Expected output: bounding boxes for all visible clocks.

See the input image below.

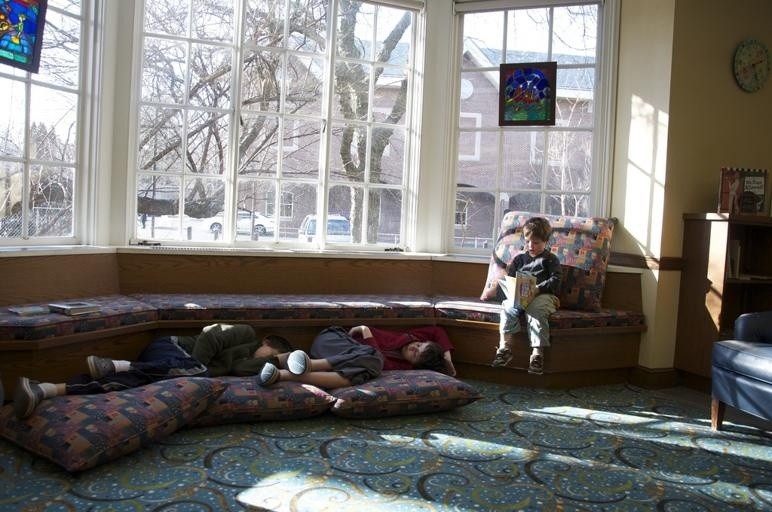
[731,40,771,94]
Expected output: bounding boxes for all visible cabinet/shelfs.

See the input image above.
[669,208,771,395]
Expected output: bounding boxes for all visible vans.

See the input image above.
[297,213,351,243]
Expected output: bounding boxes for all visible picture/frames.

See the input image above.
[1,0,51,75]
[496,60,558,127]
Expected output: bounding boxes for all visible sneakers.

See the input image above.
[256,361,280,387]
[14,376,45,418]
[85,354,116,380]
[527,353,545,375]
[490,347,514,368]
[286,349,310,376]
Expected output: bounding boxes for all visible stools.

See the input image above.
[703,307,771,437]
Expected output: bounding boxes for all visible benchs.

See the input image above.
[1,252,159,405]
[128,253,435,357]
[434,258,648,395]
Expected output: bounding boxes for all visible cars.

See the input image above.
[203,211,276,236]
[152,214,191,231]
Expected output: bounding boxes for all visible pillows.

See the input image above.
[0,365,229,478]
[321,360,486,422]
[480,208,617,315]
[185,369,339,425]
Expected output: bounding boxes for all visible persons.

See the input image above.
[492,217,562,376]
[12,324,293,419]
[256,325,456,386]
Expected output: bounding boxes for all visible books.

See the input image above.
[48,301,102,316]
[497,271,536,311]
